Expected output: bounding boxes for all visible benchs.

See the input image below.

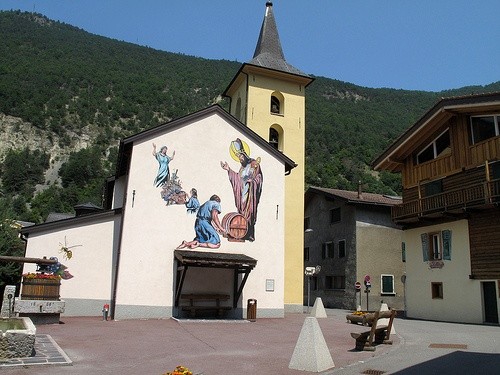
[351,308,397,351]
[181,294,232,318]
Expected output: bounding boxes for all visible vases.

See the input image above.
[21,278,62,300]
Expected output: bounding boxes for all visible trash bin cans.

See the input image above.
[247,299,257,322]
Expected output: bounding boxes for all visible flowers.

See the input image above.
[22,273,61,280]
[353,311,368,316]
[163,365,193,375]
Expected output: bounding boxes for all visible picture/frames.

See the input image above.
[266,279,274,291]
